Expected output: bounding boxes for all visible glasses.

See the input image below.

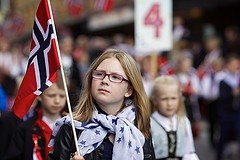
[92,69,129,83]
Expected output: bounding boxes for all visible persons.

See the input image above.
[149,74,200,160]
[223,25,240,58]
[0,5,225,111]
[5,70,70,160]
[163,51,202,139]
[50,49,156,160]
[199,54,225,149]
[213,54,240,160]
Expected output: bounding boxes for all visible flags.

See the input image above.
[62,0,85,15]
[3,11,26,36]
[11,0,62,120]
[93,0,114,12]
